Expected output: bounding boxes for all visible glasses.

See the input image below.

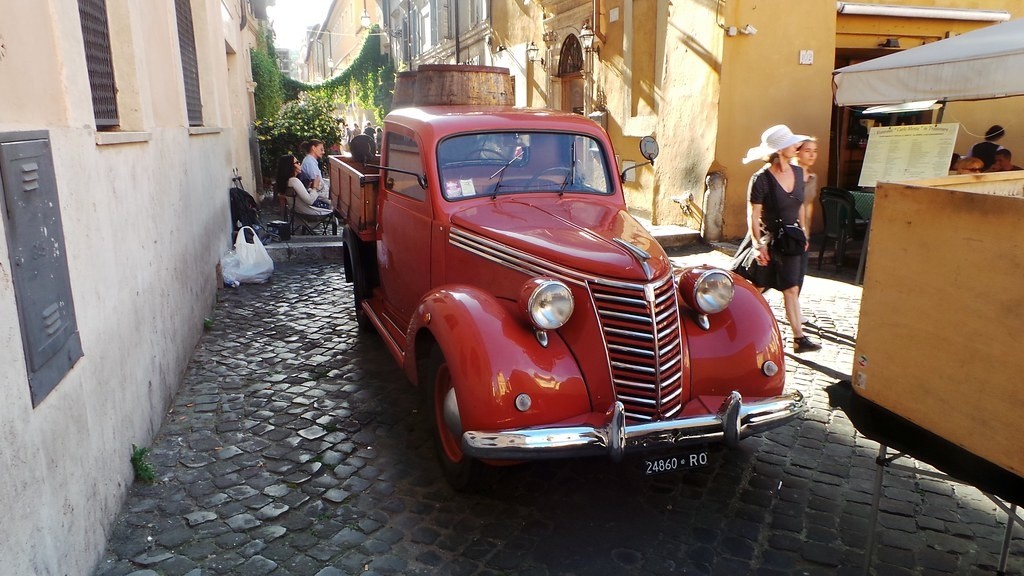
[294,160,299,164]
[963,168,984,172]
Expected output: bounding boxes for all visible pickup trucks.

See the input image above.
[326,105,809,498]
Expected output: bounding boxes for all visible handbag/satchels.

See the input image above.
[234,226,274,284]
[773,225,807,256]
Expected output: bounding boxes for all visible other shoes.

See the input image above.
[801,316,809,323]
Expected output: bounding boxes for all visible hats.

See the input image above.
[742,124,809,165]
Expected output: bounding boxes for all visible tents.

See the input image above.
[831,18,1024,107]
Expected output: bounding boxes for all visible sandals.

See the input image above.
[794,336,822,353]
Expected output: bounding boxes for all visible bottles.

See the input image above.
[222,251,240,287]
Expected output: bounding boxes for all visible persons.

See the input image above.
[274,139,350,228]
[341,122,383,155]
[949,125,1024,173]
[728,125,823,353]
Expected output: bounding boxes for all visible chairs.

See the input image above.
[272,184,337,236]
[818,186,872,286]
[849,189,875,285]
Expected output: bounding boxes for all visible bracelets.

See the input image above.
[312,187,318,190]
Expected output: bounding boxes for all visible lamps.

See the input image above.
[328,56,344,70]
[877,39,900,48]
[525,39,543,66]
[576,23,600,62]
[485,33,506,54]
[361,11,371,30]
[836,1,1011,22]
[317,73,325,82]
